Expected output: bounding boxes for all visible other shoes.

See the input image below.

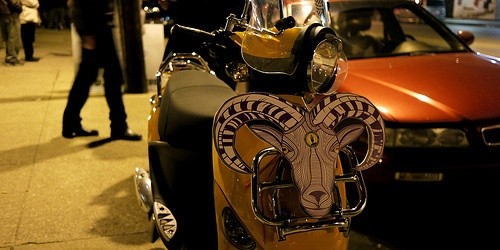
[25,57,39,61]
[5,58,23,65]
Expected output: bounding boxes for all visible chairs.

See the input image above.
[335,8,390,60]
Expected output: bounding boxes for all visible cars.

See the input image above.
[285,0,500,244]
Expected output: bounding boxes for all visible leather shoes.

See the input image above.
[62,126,98,137]
[111,129,141,140]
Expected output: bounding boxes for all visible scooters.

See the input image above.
[135,0,386,250]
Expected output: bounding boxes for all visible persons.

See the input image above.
[19,0,42,63]
[4,0,23,67]
[61,1,143,143]
